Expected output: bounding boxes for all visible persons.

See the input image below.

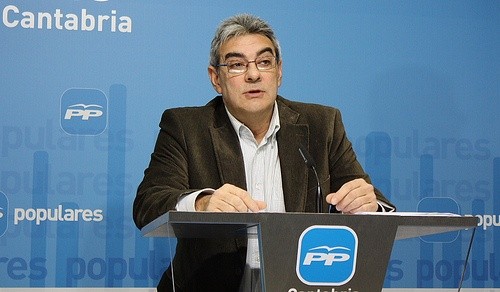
[132,12,398,292]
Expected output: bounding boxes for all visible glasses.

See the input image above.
[215,55,279,74]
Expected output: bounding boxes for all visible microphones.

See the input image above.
[298,145,314,167]
